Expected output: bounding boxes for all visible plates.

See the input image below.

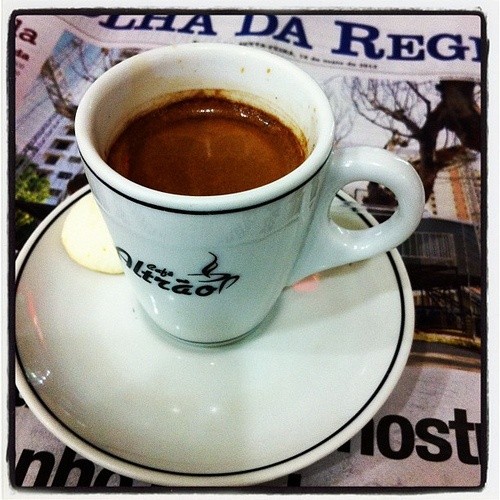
[14,183,416,487]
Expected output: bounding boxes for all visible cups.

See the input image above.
[73,43,425,349]
